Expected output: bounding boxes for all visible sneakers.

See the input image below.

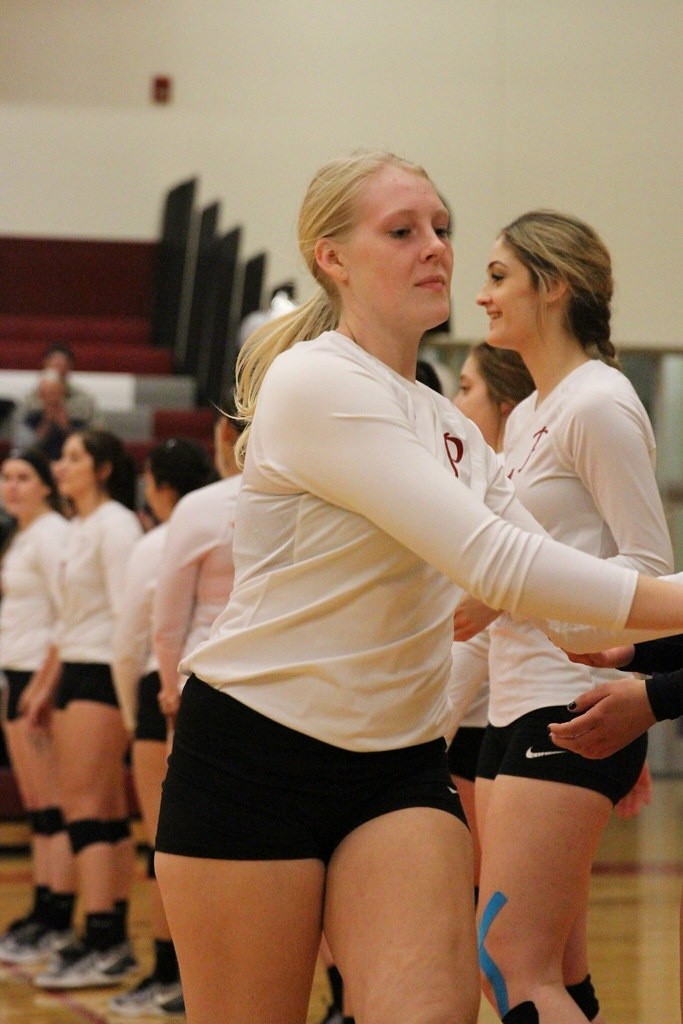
[0,919,81,967]
[112,979,185,1018]
[31,938,138,990]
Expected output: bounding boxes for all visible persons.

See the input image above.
[154,153,682,1024]
[546,633,683,759]
[11,344,103,459]
[415,213,672,1024]
[0,390,246,1014]
